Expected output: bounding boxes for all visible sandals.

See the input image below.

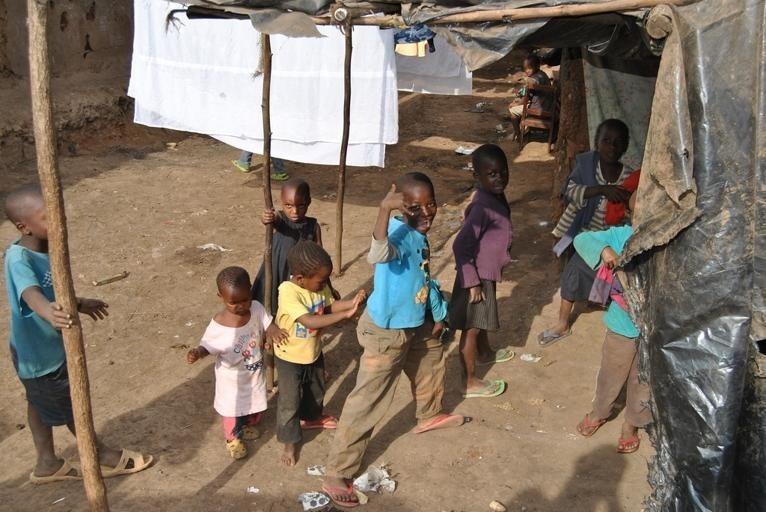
[231,160,250,172]
[270,173,289,181]
[226,437,248,459]
[239,425,260,440]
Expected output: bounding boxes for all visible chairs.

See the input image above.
[519,82,558,153]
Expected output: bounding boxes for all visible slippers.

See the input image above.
[100,447,153,478]
[29,458,84,485]
[576,414,606,436]
[321,483,359,507]
[539,330,572,348]
[618,433,639,452]
[475,349,514,367]
[300,414,338,429]
[414,413,465,433]
[462,378,505,398]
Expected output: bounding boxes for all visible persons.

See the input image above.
[230,150,292,181]
[537,118,638,349]
[321,170,465,508]
[447,145,515,398]
[572,188,656,453]
[507,55,554,142]
[186,265,292,461]
[2,184,154,485]
[249,179,342,385]
[272,239,368,467]
